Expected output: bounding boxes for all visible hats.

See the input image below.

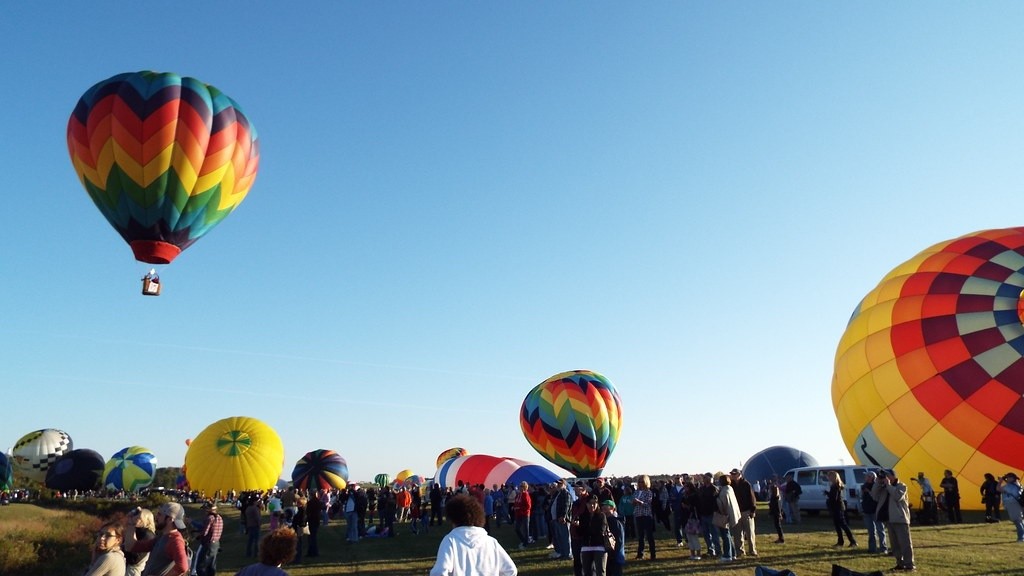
[702,473,712,478]
[161,502,186,529]
[863,470,877,479]
[556,479,566,485]
[597,478,605,483]
[730,469,739,474]
[571,481,586,487]
[201,501,214,509]
[879,469,896,477]
[601,500,616,508]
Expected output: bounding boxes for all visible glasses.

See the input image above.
[96,532,117,538]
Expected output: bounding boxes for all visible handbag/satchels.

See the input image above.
[600,513,616,552]
[1010,484,1024,507]
[712,503,728,528]
[685,507,703,535]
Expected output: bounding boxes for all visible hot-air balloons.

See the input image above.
[9,428,73,487]
[0,451,14,493]
[520,370,623,479]
[67,71,259,295]
[177,417,288,501]
[742,446,818,491]
[432,454,576,501]
[831,227,1024,511]
[436,448,467,470]
[375,474,390,488]
[390,470,425,488]
[45,449,104,493]
[102,446,156,496]
[347,481,360,490]
[292,449,348,490]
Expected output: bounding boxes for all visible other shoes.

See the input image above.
[889,565,916,572]
[835,542,843,547]
[516,536,573,560]
[674,542,757,564]
[775,538,784,543]
[848,542,858,548]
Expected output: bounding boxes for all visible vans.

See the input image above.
[782,465,881,516]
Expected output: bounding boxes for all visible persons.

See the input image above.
[940,470,962,523]
[997,474,1024,542]
[227,479,532,561]
[753,474,802,543]
[85,503,189,576]
[861,469,916,571]
[161,487,222,503]
[0,486,42,501]
[234,527,297,576]
[430,494,518,576]
[528,469,757,576]
[823,472,858,548]
[980,474,1001,519]
[51,488,150,501]
[917,474,938,520]
[198,502,223,576]
